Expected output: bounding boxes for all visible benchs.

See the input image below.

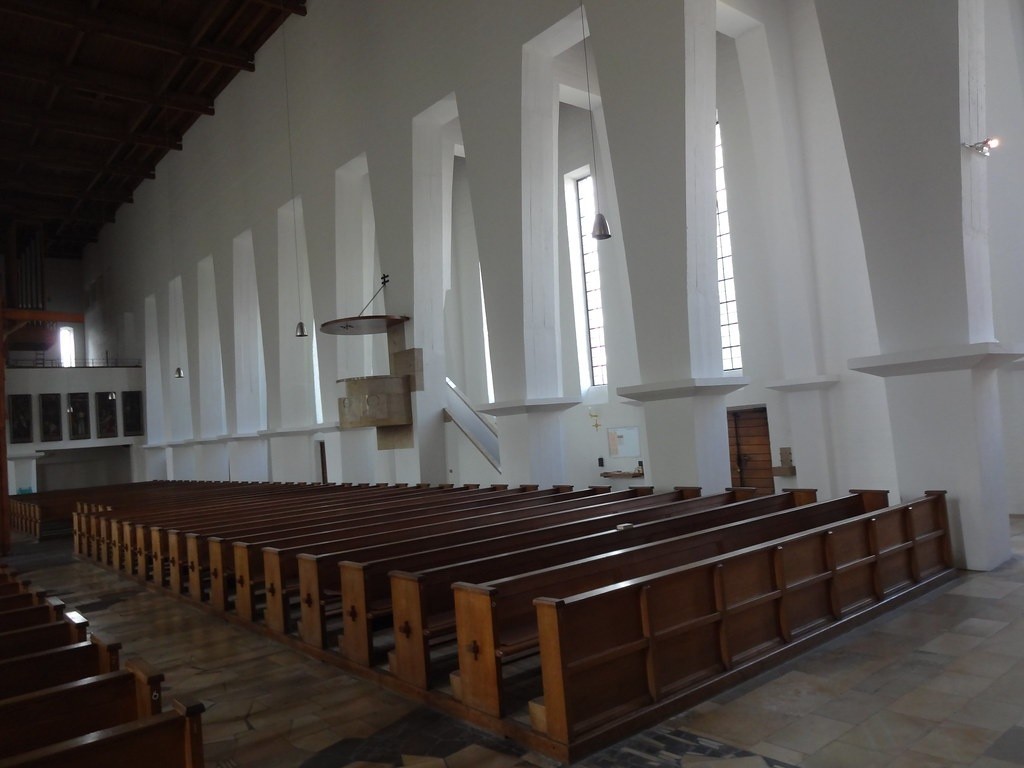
[0,479,960,768]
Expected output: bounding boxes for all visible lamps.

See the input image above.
[67,406,73,413]
[174,368,184,379]
[296,323,307,336]
[109,392,116,399]
[580,0,611,240]
